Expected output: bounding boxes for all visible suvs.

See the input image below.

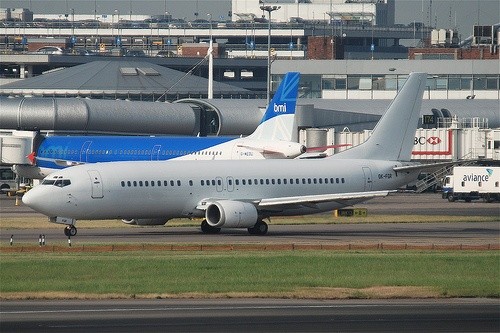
[122,49,146,57]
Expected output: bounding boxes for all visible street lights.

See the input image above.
[260,5,281,109]
[165,11,170,44]
[113,9,120,45]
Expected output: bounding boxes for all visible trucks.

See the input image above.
[440,166,500,203]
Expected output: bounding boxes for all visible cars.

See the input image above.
[188,18,209,28]
[154,50,175,57]
[31,45,95,56]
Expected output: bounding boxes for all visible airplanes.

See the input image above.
[8,71,308,181]
[21,71,494,237]
